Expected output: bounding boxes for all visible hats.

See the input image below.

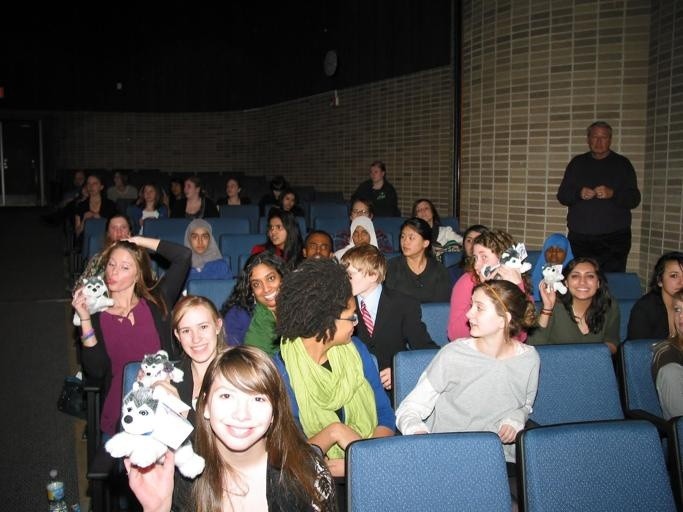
[270,175,289,191]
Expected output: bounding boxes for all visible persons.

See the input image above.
[270,257,395,511]
[71,236,191,445]
[652,289,683,421]
[124,344,338,512]
[136,294,231,421]
[392,279,540,511]
[447,224,682,350]
[52,162,244,279]
[221,163,464,396]
[556,121,642,274]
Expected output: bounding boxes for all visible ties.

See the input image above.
[360,300,373,338]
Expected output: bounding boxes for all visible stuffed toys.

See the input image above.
[104,379,204,479]
[140,350,183,383]
[73,276,115,326]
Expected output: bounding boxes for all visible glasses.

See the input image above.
[339,314,359,327]
[351,208,368,215]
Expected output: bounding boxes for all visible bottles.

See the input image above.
[45,468,69,511]
[66,489,80,512]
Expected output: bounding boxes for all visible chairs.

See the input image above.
[61,165,683,512]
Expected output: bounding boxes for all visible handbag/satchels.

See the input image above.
[58,375,86,419]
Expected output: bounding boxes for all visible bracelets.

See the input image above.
[79,317,90,322]
[78,328,95,344]
